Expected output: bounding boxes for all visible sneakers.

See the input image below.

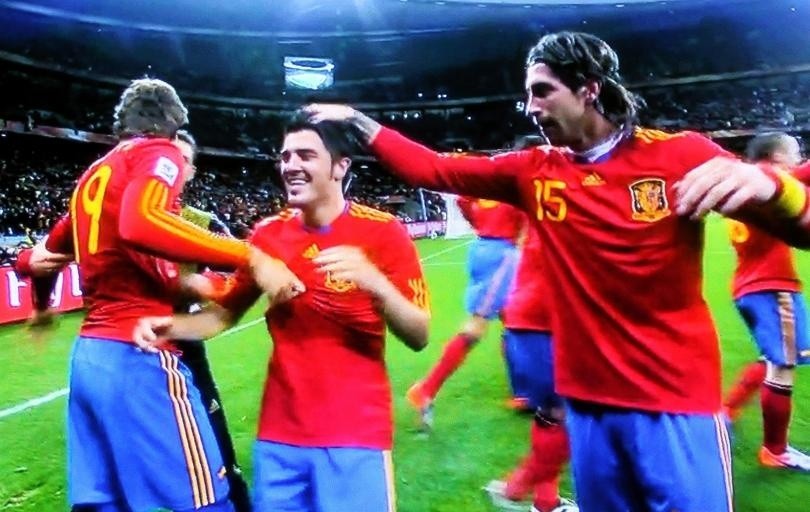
[758,441,809,471]
[529,494,579,512]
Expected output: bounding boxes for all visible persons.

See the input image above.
[0,72,115,235]
[31,78,305,511]
[309,32,810,512]
[644,87,808,163]
[407,137,577,511]
[184,137,286,238]
[343,162,447,223]
[17,131,253,512]
[722,132,809,470]
[133,109,431,512]
